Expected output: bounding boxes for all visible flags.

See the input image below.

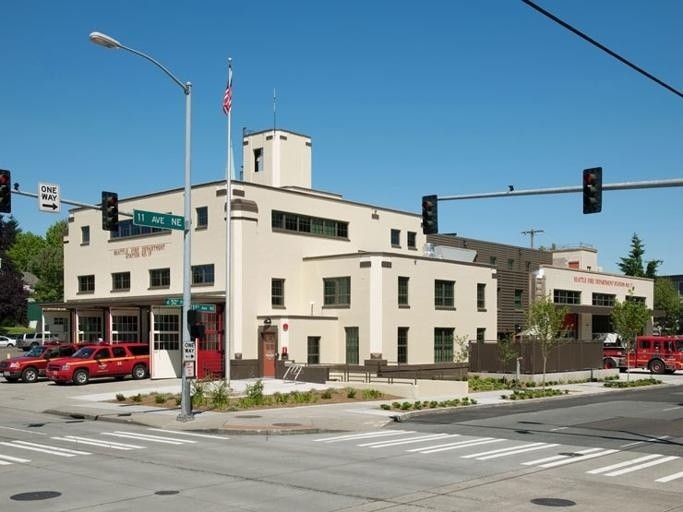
[223,64,232,116]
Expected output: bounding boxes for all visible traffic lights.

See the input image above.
[102,191,118,231]
[583,167,602,214]
[0,169,10,213]
[422,195,438,234]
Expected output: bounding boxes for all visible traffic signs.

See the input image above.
[38,182,60,213]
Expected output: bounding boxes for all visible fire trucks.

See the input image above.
[603,335,683,374]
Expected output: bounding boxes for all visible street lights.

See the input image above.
[89,32,192,416]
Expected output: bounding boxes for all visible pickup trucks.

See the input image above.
[0,331,149,385]
